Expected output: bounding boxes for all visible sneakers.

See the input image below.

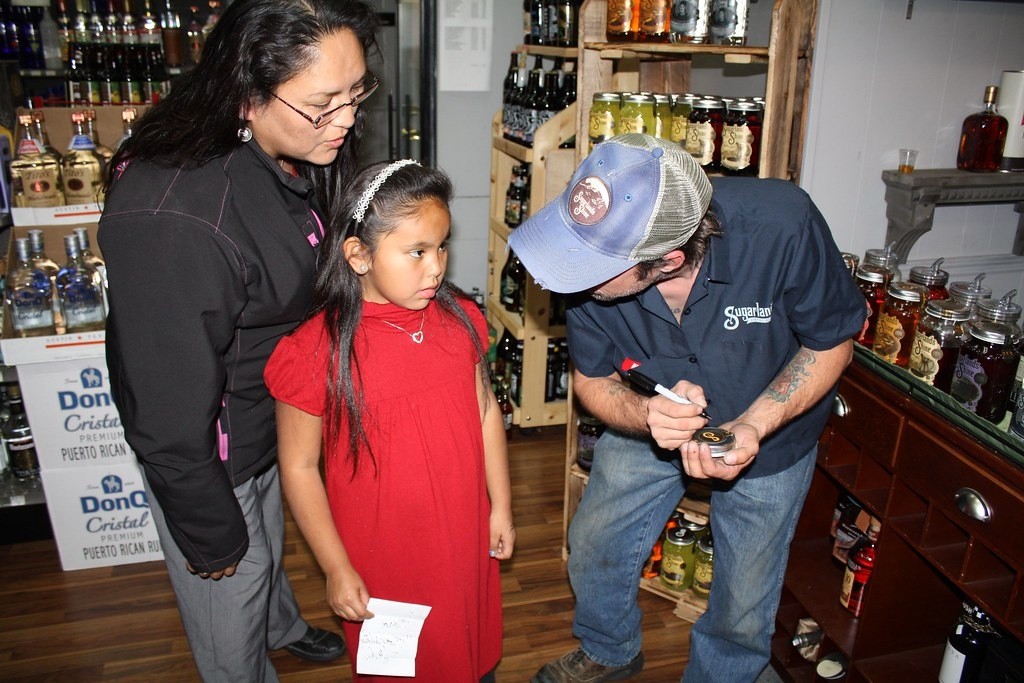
[530,646,645,683]
[286,625,346,662]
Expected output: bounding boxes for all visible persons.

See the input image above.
[96,0,386,682]
[263,159,516,683]
[508,132,867,683]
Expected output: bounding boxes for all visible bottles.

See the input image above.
[829,493,883,622]
[0,0,219,340]
[576,414,714,599]
[484,0,767,440]
[0,381,40,477]
[956,86,1008,174]
[938,605,1002,683]
[837,248,1024,441]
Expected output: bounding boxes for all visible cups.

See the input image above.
[899,148,918,174]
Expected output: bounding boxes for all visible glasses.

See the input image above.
[261,71,381,130]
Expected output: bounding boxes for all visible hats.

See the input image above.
[506,132,715,295]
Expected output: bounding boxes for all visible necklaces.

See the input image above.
[382,311,425,344]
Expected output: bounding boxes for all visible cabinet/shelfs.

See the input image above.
[0,0,1024,683]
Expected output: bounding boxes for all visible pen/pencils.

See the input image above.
[626,369,711,419]
